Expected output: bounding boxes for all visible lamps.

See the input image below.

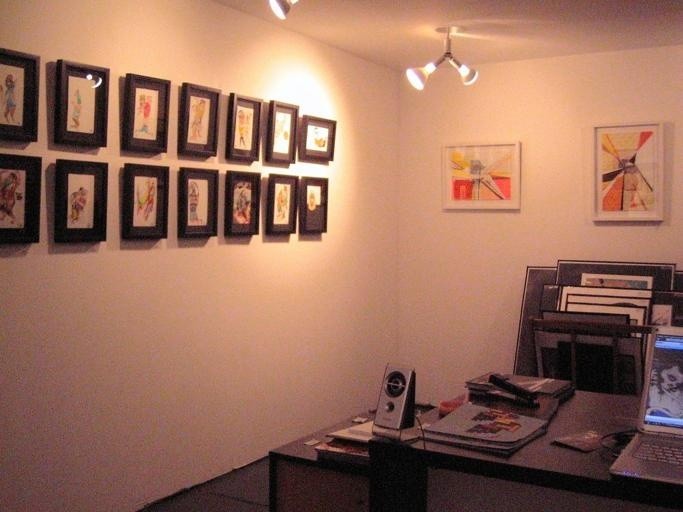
[403,24,478,90]
[267,0,302,19]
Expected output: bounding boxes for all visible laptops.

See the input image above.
[609,325,683,486]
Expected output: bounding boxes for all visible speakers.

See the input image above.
[372,363,418,441]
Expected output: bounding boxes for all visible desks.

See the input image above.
[266,406,435,512]
[366,375,682,511]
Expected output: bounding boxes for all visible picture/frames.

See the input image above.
[56,59,109,146]
[266,101,298,162]
[180,82,220,155]
[300,115,332,160]
[224,170,262,235]
[0,46,40,142]
[2,154,41,243]
[515,258,683,395]
[123,74,170,152]
[227,93,262,161]
[123,163,168,238]
[55,159,108,243]
[440,141,521,210]
[300,175,329,233]
[267,173,298,233]
[591,120,664,222]
[178,167,219,236]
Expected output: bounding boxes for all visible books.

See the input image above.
[465,372,577,408]
[314,420,375,465]
